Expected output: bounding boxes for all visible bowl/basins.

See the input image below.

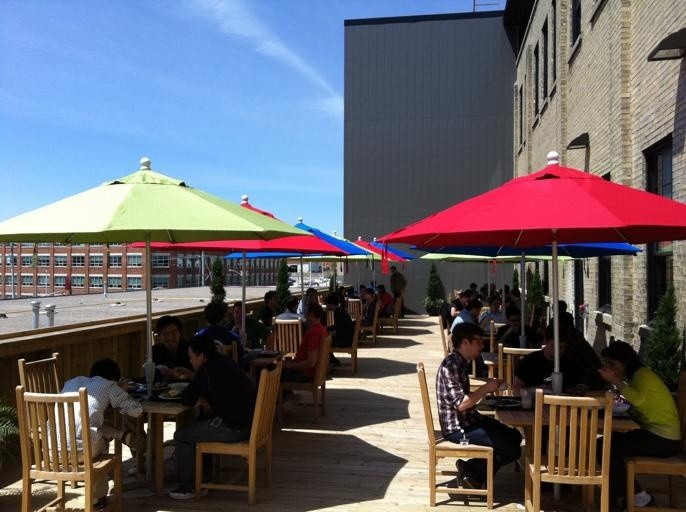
[167,382,188,391]
[612,403,630,413]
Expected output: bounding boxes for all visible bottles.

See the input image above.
[520,389,535,408]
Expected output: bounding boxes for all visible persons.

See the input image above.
[180,336,253,500]
[277,304,329,420]
[360,280,395,336]
[513,327,575,393]
[152,315,196,371]
[195,299,266,351]
[436,323,522,491]
[256,291,282,326]
[324,295,354,368]
[450,285,573,347]
[390,268,407,318]
[592,341,681,509]
[44,358,144,465]
[276,297,304,319]
[303,288,318,307]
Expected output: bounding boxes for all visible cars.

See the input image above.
[286,276,342,287]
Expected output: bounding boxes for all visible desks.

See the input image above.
[116,379,191,499]
[492,390,640,504]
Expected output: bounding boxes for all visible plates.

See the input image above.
[157,391,180,400]
[260,350,276,354]
[128,394,146,401]
[486,395,522,408]
[140,384,166,392]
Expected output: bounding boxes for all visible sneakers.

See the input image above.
[169,486,207,500]
[126,434,146,451]
[456,459,484,490]
[633,490,652,507]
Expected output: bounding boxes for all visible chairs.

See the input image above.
[524,387,615,512]
[264,286,402,369]
[418,360,495,511]
[15,384,123,512]
[627,325,685,512]
[15,352,64,440]
[418,288,686,402]
[195,361,284,504]
[278,335,332,425]
